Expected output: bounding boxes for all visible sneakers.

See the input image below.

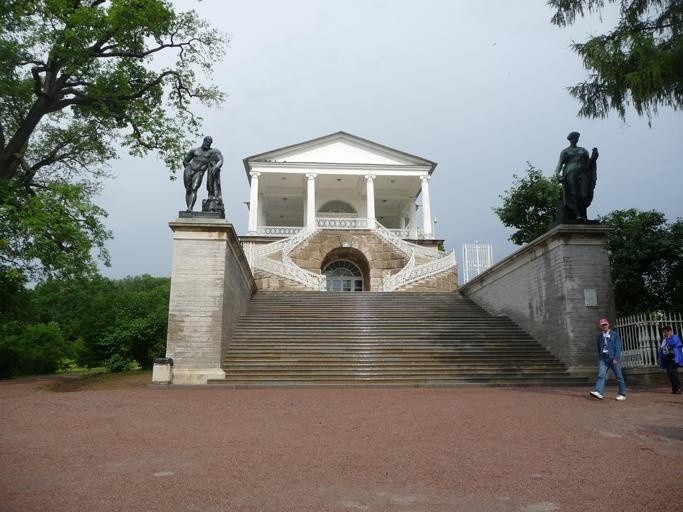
[616,394,625,400]
[590,392,603,399]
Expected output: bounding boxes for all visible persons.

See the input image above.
[556,131,599,221]
[182,136,223,212]
[657,325,683,394]
[590,317,628,400]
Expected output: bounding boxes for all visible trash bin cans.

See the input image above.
[152,358,173,384]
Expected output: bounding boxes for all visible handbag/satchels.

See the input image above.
[661,345,675,361]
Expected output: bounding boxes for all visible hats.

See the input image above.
[599,319,609,325]
